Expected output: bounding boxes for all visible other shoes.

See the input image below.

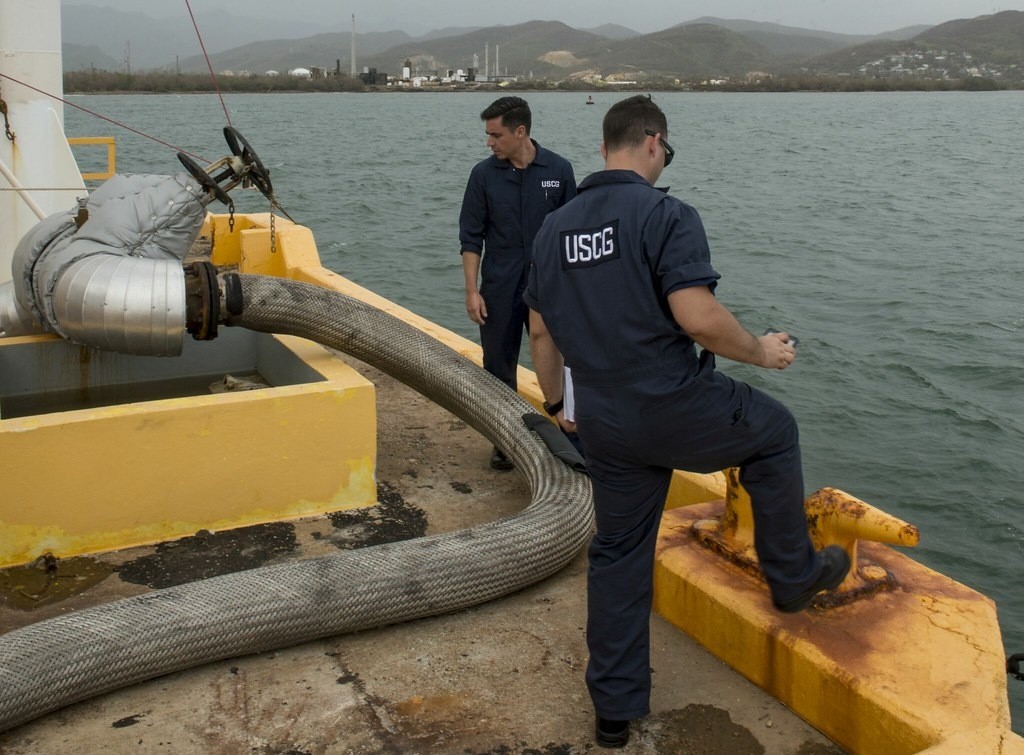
[596,712,631,748]
[774,545,851,615]
[491,444,515,469]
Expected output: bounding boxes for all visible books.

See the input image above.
[561,362,574,426]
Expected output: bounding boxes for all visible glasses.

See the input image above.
[646,128,675,167]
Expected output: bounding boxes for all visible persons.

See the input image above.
[458,96,578,471]
[521,94,853,749]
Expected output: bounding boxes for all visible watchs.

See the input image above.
[540,398,564,418]
